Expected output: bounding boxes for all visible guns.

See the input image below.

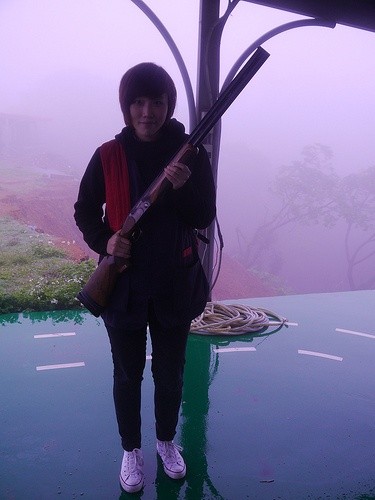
[75,45,271,318]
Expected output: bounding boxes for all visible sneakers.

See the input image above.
[118,447,145,494]
[155,436,187,479]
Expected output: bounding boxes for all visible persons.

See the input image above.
[70,60,216,495]
